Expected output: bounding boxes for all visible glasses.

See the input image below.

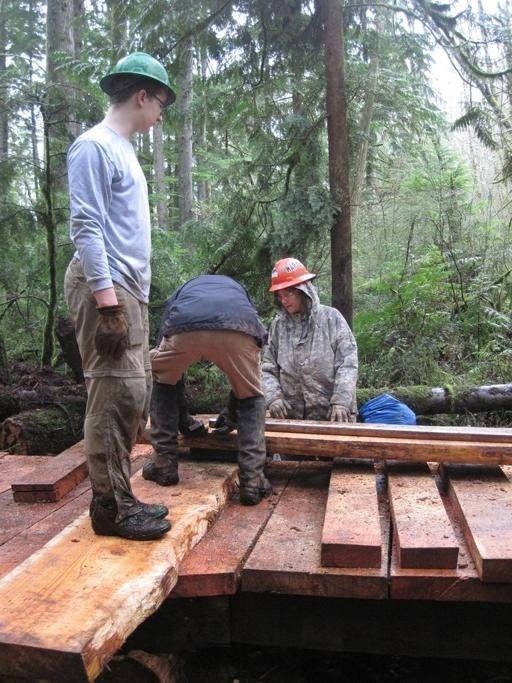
[272,289,297,298]
[154,95,169,111]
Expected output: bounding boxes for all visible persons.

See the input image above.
[262,258,358,422]
[63,51,172,539]
[143,276,272,505]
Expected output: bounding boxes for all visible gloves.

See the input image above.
[181,410,207,437]
[325,405,353,423]
[268,398,293,419]
[94,305,131,361]
[212,405,235,435]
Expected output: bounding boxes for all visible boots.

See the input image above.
[230,389,272,506]
[142,377,185,486]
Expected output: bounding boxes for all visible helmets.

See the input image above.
[99,52,176,107]
[268,258,317,292]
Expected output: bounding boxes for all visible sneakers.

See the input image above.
[89,502,171,540]
